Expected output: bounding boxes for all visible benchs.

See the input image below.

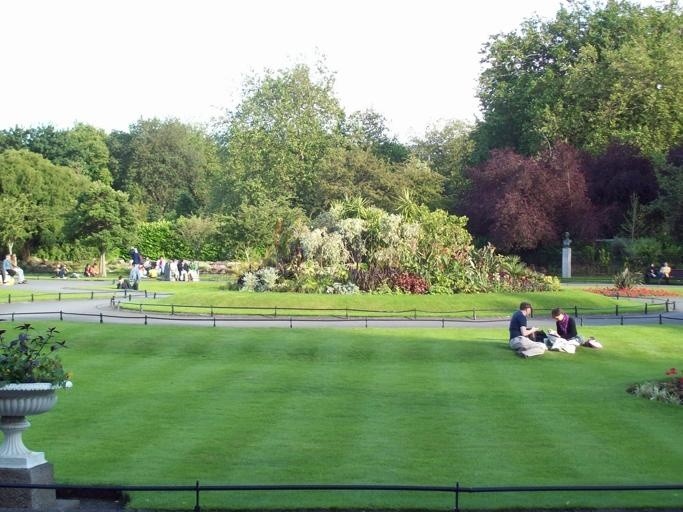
[643,268,683,286]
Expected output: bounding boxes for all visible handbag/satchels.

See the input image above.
[535,331,548,342]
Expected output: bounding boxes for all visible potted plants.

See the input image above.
[0,318,73,468]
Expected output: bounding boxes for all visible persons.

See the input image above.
[659,261,671,286]
[548,308,583,346]
[646,263,657,283]
[2,254,15,277]
[510,302,548,359]
[11,254,28,284]
[55,249,199,290]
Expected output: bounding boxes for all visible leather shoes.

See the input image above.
[515,352,527,358]
